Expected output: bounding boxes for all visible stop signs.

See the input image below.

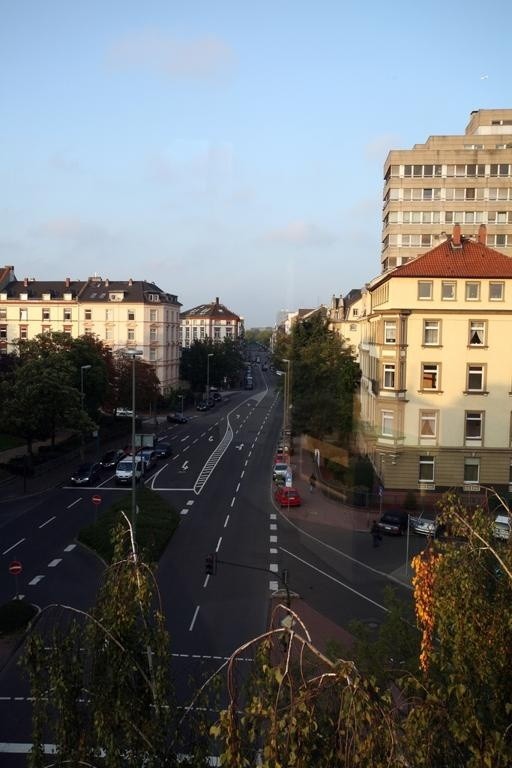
[92,495,103,504]
[9,561,22,575]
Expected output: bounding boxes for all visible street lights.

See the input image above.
[81,364,92,459]
[277,358,290,464]
[122,349,143,558]
[207,354,215,399]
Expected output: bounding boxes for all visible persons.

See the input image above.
[437,523,447,542]
[370,520,381,547]
[309,473,316,492]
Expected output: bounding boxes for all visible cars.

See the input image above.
[68,463,103,487]
[100,448,126,466]
[378,509,407,536]
[197,393,221,411]
[137,442,170,469]
[256,357,268,371]
[273,425,301,507]
[243,364,253,390]
[167,415,187,423]
[409,514,435,537]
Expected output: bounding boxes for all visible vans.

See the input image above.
[115,456,145,484]
[493,515,512,540]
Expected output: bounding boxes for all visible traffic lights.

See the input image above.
[206,553,217,576]
